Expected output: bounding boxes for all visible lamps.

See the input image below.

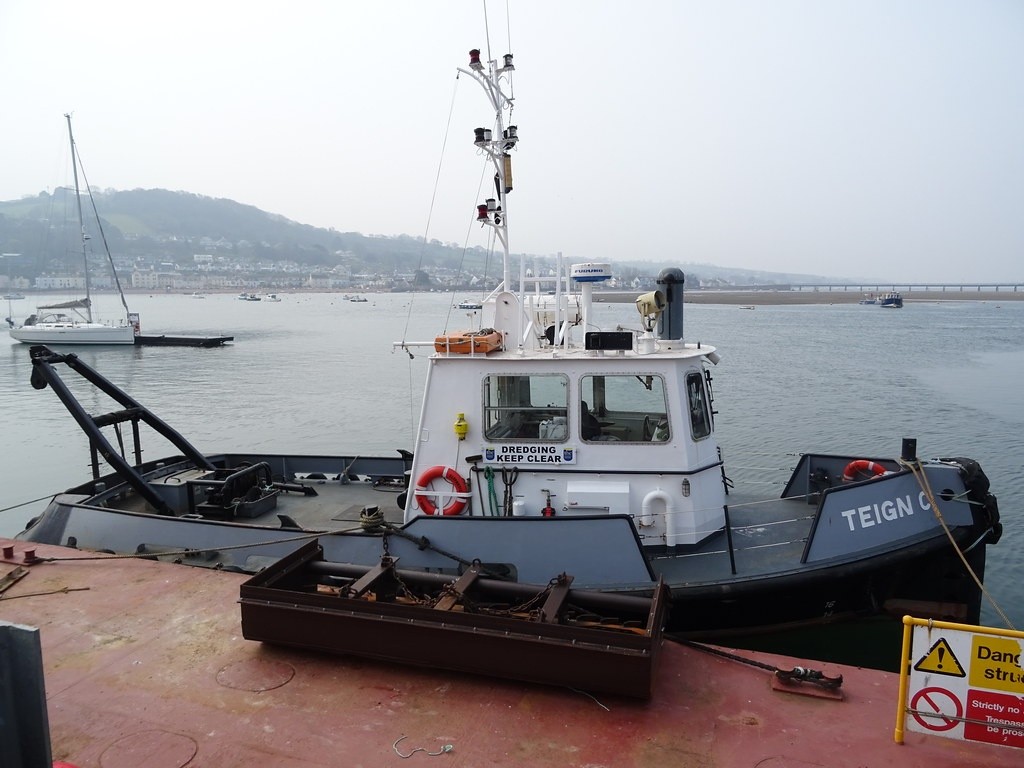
[636,290,667,332]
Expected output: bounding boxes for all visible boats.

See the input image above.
[458,298,481,310]
[238,288,282,304]
[349,297,368,303]
[858,294,878,305]
[7,2,1004,675]
[879,287,904,309]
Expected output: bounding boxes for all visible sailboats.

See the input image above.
[3,111,237,348]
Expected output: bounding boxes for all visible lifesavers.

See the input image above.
[415,466,468,516]
[842,460,887,482]
